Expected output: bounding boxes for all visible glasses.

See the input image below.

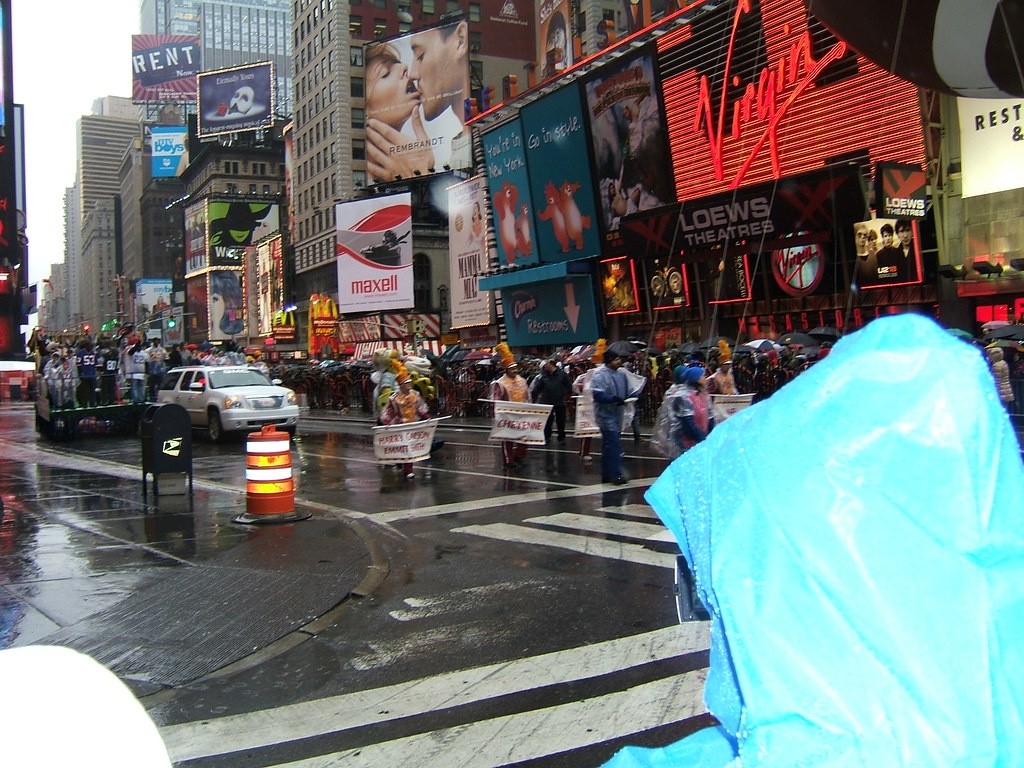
[856,232,868,239]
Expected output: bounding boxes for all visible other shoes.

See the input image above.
[406,473,415,478]
[601,475,626,485]
[579,456,593,461]
[393,466,397,468]
[50,398,158,411]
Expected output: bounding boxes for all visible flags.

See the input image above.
[20,283,39,314]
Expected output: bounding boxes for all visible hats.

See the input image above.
[820,341,832,347]
[396,373,412,384]
[603,349,619,366]
[717,355,733,364]
[102,339,113,347]
[128,337,140,345]
[187,344,200,351]
[46,342,59,351]
[495,343,518,368]
[209,348,224,354]
[592,353,603,364]
[66,350,73,353]
[200,339,213,351]
[674,365,704,384]
[152,338,160,343]
[253,350,263,357]
[246,356,255,361]
[779,346,789,353]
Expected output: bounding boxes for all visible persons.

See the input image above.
[854,219,917,284]
[599,313,1023,768]
[365,21,469,180]
[608,92,658,214]
[471,202,481,235]
[153,296,169,313]
[38,333,1024,492]
[210,270,243,338]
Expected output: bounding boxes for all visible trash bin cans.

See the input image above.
[140,403,194,497]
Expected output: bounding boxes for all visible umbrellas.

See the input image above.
[428,321,1024,367]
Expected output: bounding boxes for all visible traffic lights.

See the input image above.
[167,313,178,330]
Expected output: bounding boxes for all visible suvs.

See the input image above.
[155,364,301,445]
